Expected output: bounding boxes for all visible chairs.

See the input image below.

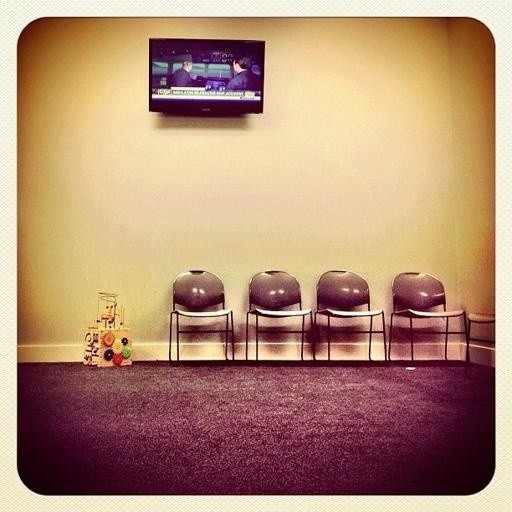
[245,271,316,368]
[169,271,235,369]
[313,272,388,370]
[466,312,495,372]
[388,272,471,368]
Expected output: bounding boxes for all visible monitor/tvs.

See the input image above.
[149,38,265,115]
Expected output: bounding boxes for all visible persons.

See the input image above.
[171,60,193,87]
[227,56,261,91]
